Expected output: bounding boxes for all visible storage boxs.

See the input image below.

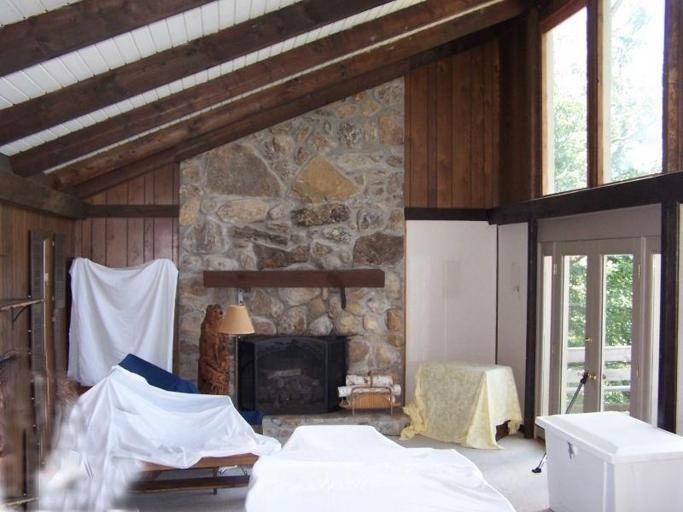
[535,411,683,512]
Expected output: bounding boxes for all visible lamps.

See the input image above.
[215,304,255,412]
